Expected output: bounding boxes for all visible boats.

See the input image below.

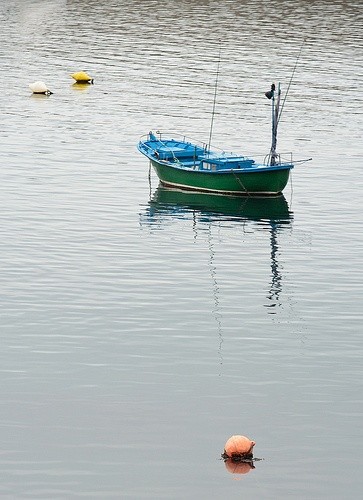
[142,187,293,313]
[139,39,313,198]
